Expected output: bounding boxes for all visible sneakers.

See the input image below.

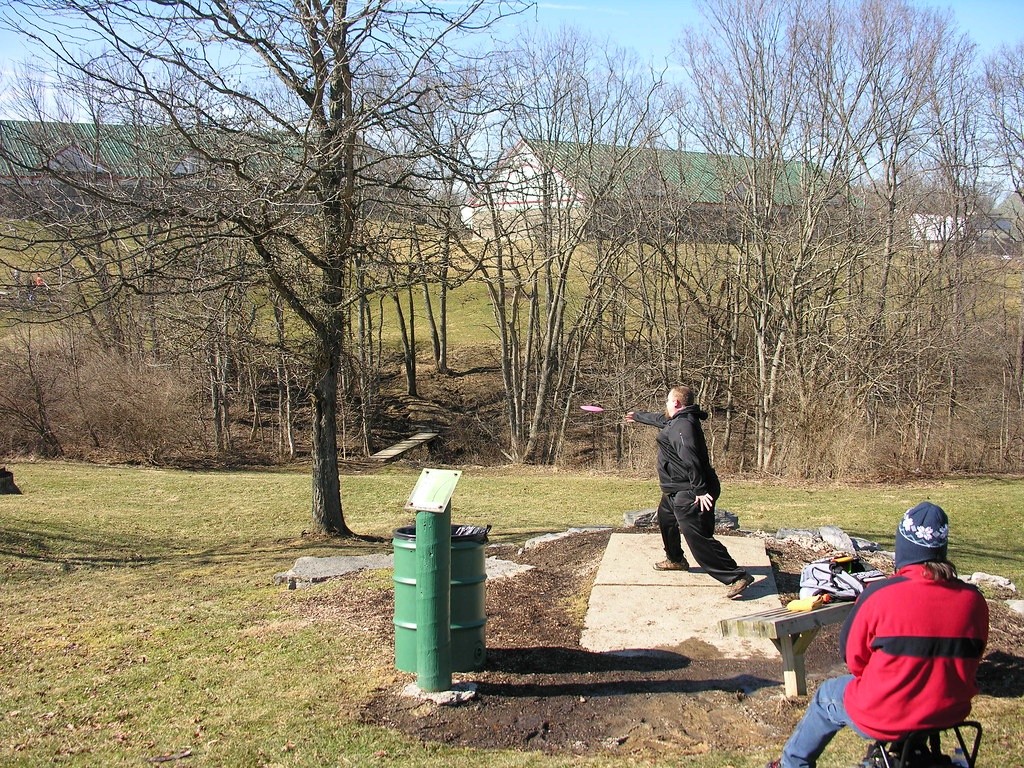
[653,557,689,571]
[727,573,755,598]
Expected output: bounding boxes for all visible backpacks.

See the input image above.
[799,555,887,602]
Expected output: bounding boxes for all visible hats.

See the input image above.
[895,501,948,569]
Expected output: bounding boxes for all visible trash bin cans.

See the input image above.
[392,527,490,670]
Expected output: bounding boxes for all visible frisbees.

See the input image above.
[580,405,604,412]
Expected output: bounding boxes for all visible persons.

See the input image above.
[765,501,989,768]
[625,386,755,598]
